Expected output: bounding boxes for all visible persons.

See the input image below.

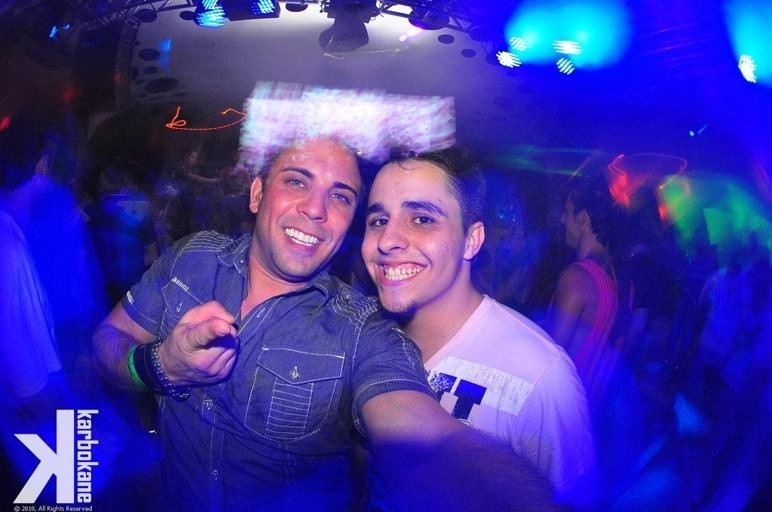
[0,118,770,510]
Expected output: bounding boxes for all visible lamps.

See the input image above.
[318,0,384,58]
